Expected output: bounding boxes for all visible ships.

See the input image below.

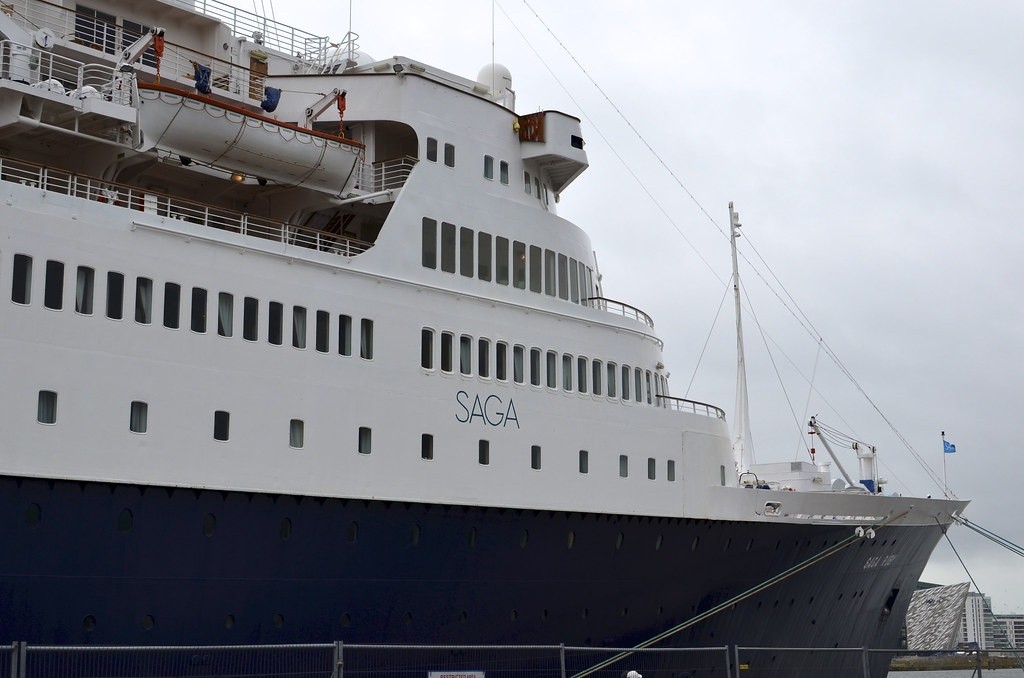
[1,3,975,678]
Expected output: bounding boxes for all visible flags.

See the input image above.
[944,441,956,453]
